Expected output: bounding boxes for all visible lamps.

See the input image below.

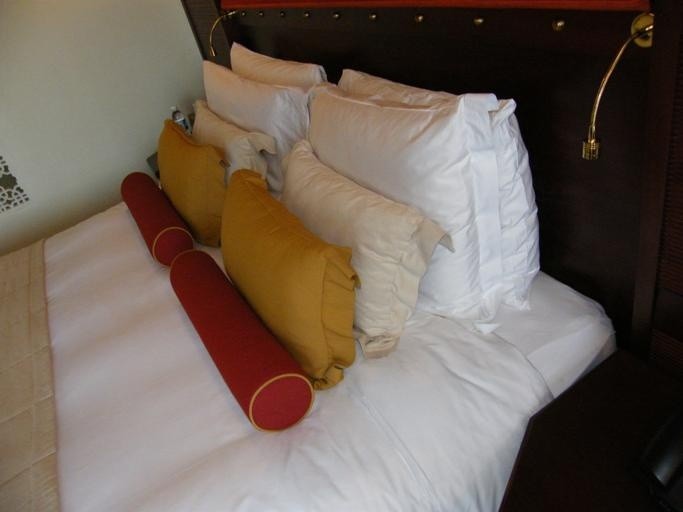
[202,8,237,58]
[575,11,655,163]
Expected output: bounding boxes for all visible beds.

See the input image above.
[0,3,676,512]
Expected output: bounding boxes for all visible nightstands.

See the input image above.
[501,342,679,512]
[144,149,161,182]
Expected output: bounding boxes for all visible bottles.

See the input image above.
[169,105,191,136]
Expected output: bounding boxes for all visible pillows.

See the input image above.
[219,168,362,395]
[200,56,308,191]
[305,80,482,322]
[165,247,315,433]
[152,118,234,247]
[332,65,546,323]
[188,95,278,181]
[118,168,195,268]
[228,39,327,96]
[275,136,458,354]
